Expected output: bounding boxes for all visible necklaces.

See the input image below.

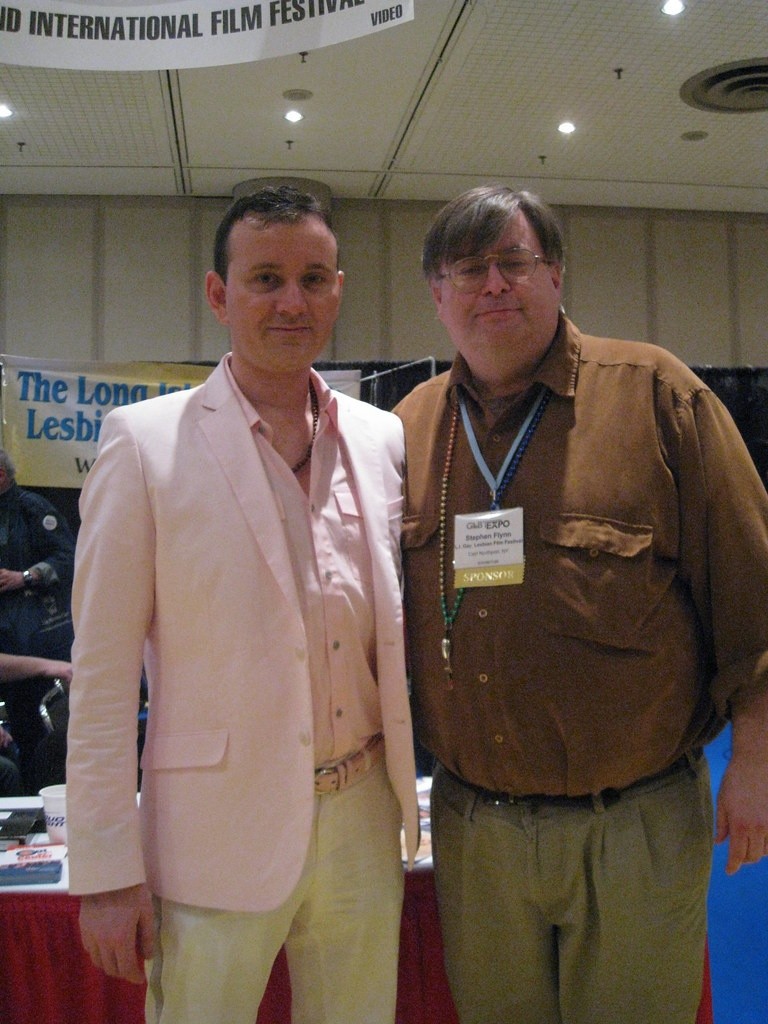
[439,389,552,690]
[290,378,317,473]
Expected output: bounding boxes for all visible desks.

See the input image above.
[0,781,712,1024]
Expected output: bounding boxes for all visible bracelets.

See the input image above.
[23,570,32,585]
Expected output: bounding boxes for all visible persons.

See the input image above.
[0,450,76,795]
[395,185,768,1024]
[0,653,72,797]
[69,186,422,1023]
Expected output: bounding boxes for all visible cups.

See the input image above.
[39,783,67,845]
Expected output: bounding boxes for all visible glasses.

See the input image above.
[438,249,552,295]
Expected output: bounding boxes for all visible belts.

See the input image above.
[437,742,704,811]
[315,733,386,798]
